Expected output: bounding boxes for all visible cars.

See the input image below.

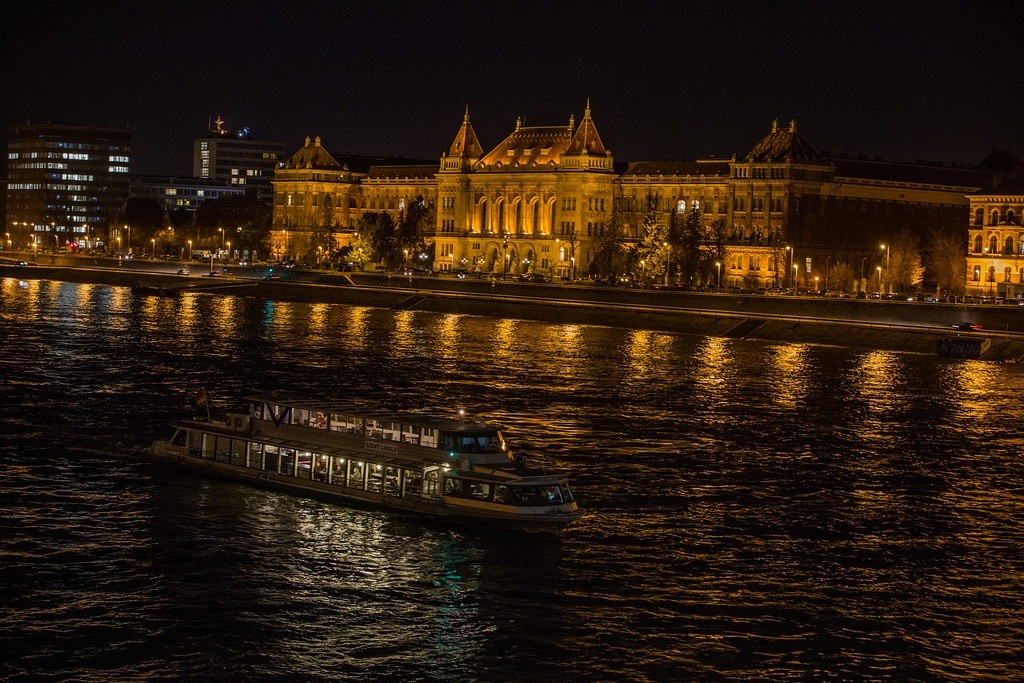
[270,258,1023,310]
[954,323,980,332]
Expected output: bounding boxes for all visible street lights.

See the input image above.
[571,257,574,281]
[188,240,192,258]
[794,264,799,293]
[218,227,224,249]
[404,249,408,274]
[880,244,890,293]
[355,231,361,253]
[282,229,289,260]
[419,253,428,273]
[4,224,91,253]
[664,242,671,276]
[462,258,468,275]
[715,263,720,291]
[877,266,882,300]
[117,237,121,250]
[168,227,174,248]
[786,245,793,287]
[226,242,231,256]
[151,239,155,257]
[984,239,995,304]
[640,260,645,273]
[522,258,531,277]
[124,224,131,250]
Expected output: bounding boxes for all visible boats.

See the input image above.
[143,388,588,537]
